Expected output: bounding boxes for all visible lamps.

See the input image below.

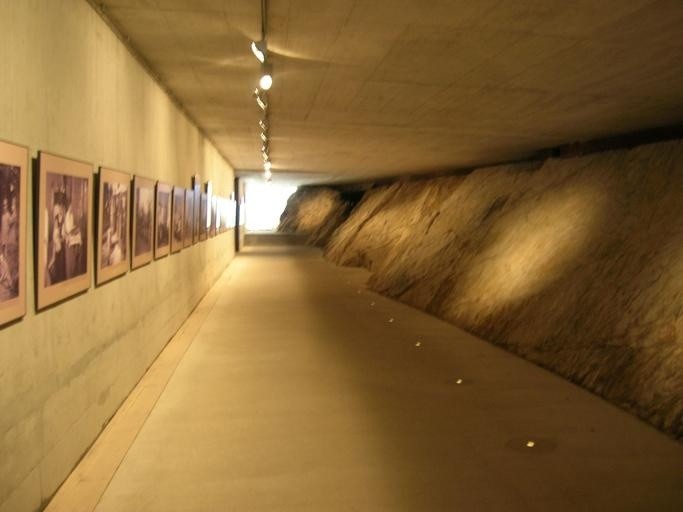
[250,40,273,181]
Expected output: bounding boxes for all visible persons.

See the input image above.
[1,195,11,252]
[47,191,81,286]
[6,196,19,284]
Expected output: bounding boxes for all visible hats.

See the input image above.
[54,191,66,207]
